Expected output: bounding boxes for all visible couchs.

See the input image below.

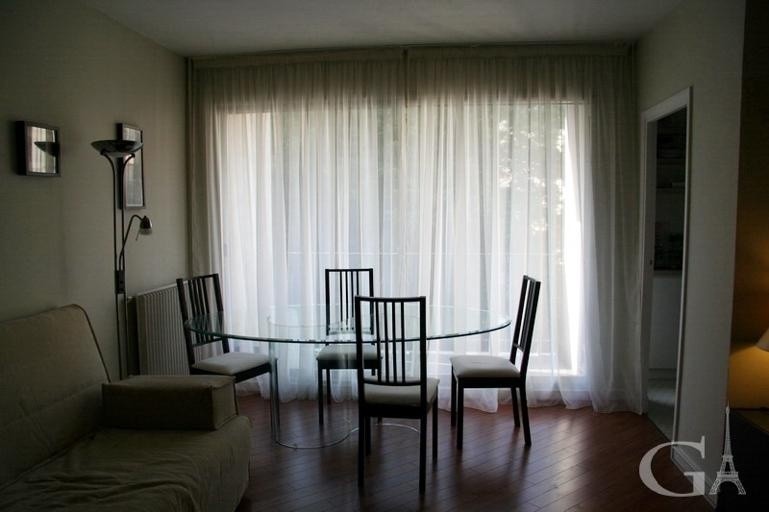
[1,303,249,512]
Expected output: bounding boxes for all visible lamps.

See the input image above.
[90,140,153,379]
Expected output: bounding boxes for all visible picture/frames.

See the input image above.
[21,120,62,177]
[117,123,147,210]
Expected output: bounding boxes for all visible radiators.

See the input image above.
[127,279,205,374]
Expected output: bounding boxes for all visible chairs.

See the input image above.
[176,269,543,499]
